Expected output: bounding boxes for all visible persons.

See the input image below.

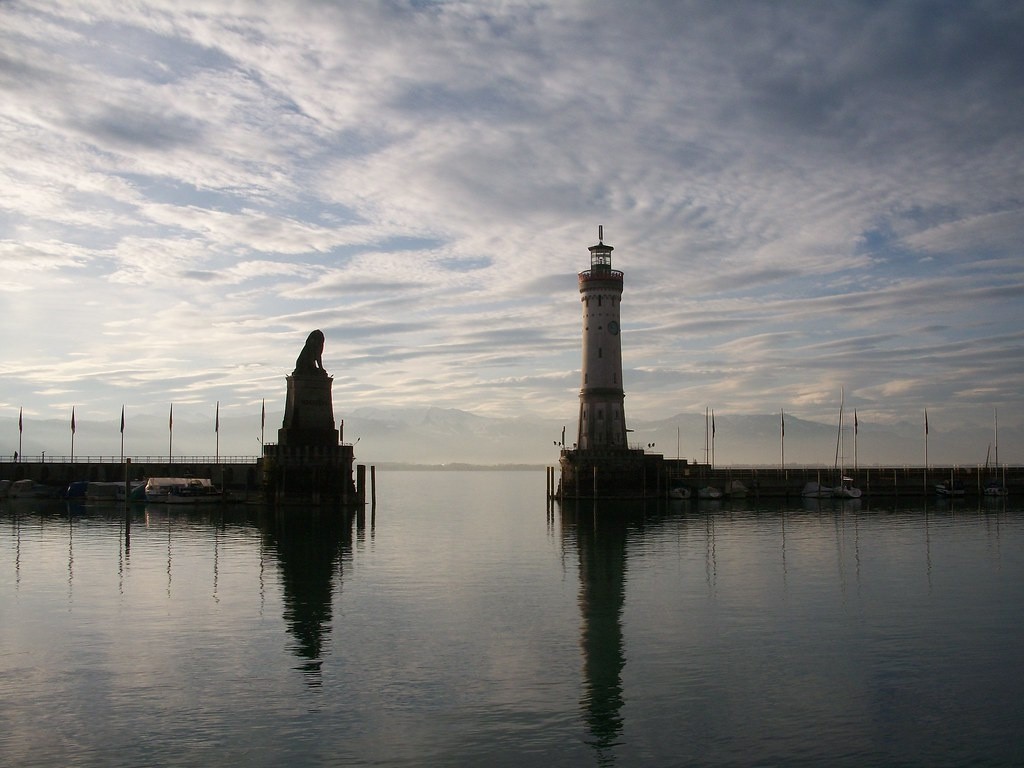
[14,451,18,461]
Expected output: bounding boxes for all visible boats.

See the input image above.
[1,479,12,499]
[670,478,692,500]
[9,478,49,498]
[801,481,834,498]
[698,484,723,501]
[984,484,1009,497]
[722,480,750,499]
[66,476,236,503]
[934,484,967,496]
[835,485,862,498]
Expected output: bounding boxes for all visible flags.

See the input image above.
[120,405,124,434]
[71,406,75,435]
[19,408,22,433]
